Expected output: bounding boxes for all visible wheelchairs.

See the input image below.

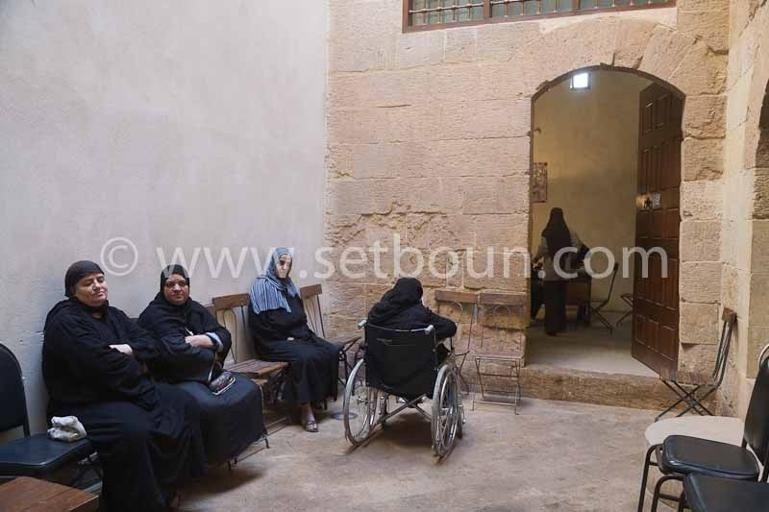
[339,312,466,456]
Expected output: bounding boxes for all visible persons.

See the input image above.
[532,207,579,336]
[248,247,338,432]
[367,278,457,409]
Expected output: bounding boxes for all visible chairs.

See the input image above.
[582,262,620,334]
[637,306,768,512]
[0,282,364,512]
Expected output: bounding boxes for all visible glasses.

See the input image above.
[278,259,293,266]
[165,279,188,287]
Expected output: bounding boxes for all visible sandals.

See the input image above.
[300,409,320,433]
